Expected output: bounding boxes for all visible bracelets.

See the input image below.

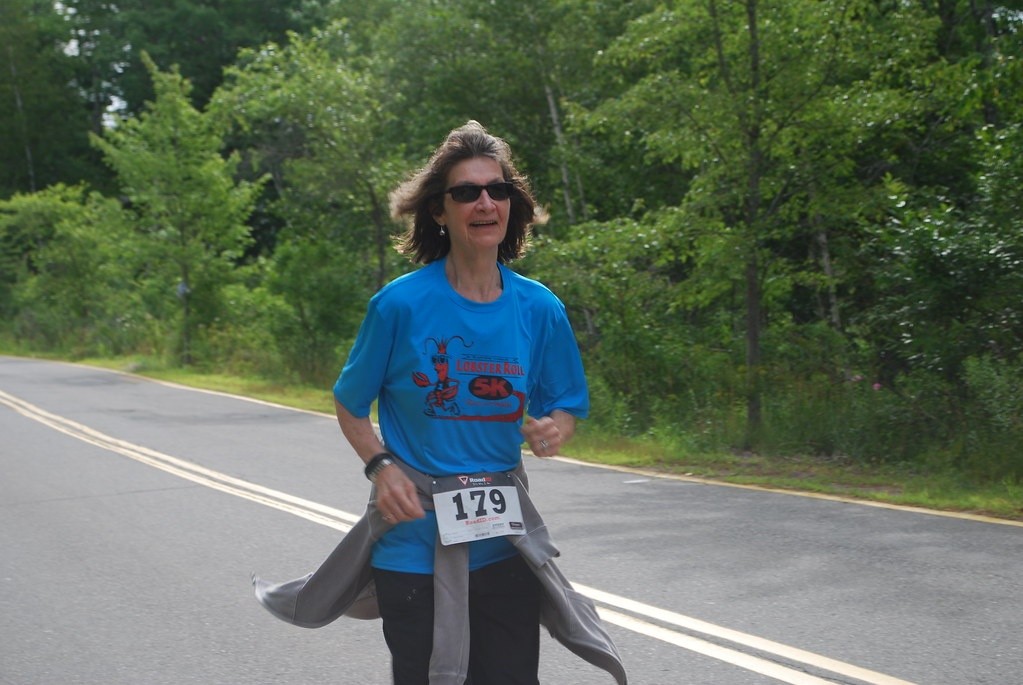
[365,453,394,483]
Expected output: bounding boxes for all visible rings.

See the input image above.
[540,440,549,449]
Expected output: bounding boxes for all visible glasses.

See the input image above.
[443,182,514,203]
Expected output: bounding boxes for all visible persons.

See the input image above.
[332,119,591,685]
